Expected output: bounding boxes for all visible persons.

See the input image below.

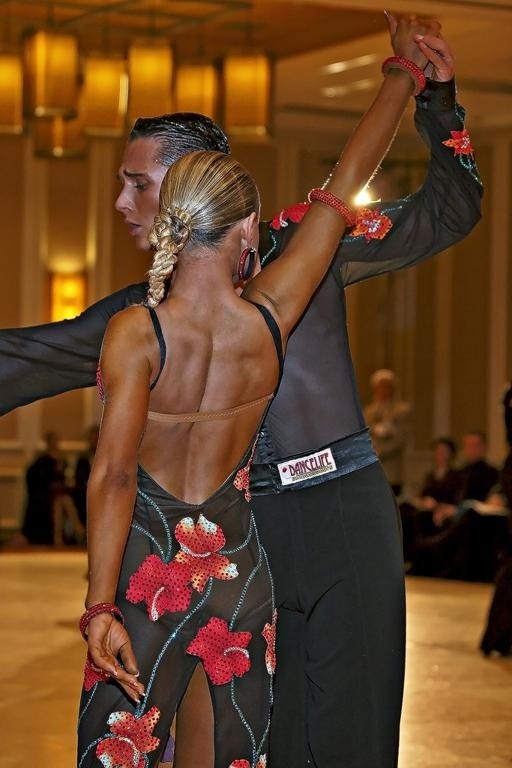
[23,426,85,547]
[480,390,511,660]
[76,8,443,768]
[395,438,465,573]
[73,426,101,528]
[362,366,414,503]
[0,29,488,766]
[414,478,512,584]
[405,431,500,578]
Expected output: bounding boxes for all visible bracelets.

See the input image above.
[382,55,424,98]
[81,600,128,638]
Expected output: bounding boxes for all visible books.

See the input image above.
[458,499,509,518]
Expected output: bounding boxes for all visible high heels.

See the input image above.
[479,637,512,657]
